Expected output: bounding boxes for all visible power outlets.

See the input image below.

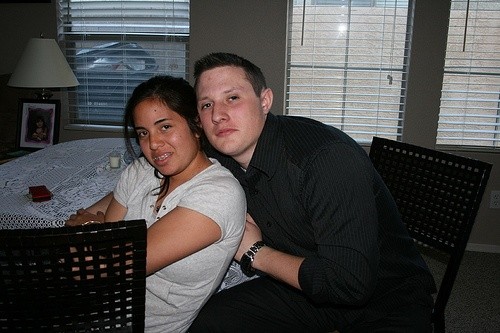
[490,190,500,209]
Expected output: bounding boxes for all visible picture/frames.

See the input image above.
[16,99,61,151]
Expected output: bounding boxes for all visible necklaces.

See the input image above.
[156,206,160,213]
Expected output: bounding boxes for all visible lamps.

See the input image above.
[7,31,81,98]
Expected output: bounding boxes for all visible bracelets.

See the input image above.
[82,220,101,226]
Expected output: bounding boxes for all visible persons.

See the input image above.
[32,116,50,141]
[67,51,384,332]
[64,75,247,333]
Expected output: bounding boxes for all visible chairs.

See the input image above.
[367,136,493,333]
[0,219,148,333]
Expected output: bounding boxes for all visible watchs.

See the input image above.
[240,240,266,278]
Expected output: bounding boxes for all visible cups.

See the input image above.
[108,151,121,169]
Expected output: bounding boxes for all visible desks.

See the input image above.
[0,138,139,229]
